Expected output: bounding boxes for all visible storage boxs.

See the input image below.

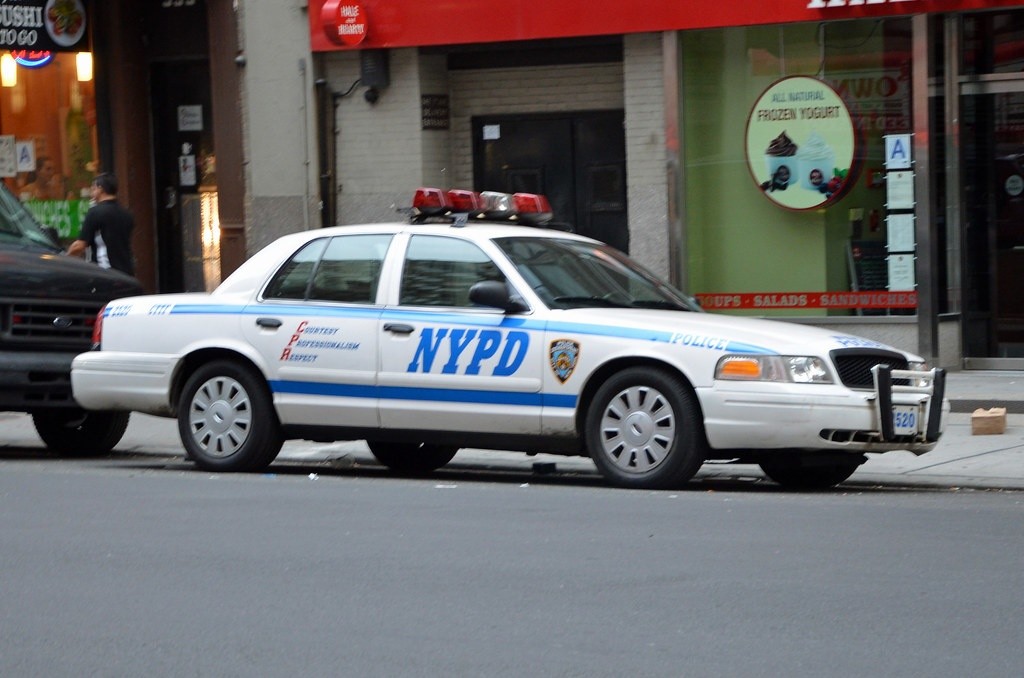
[970,405,1007,435]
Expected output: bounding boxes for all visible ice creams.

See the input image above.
[764,128,833,188]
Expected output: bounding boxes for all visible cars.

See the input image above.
[66,186,949,490]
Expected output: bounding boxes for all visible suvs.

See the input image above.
[0,178,148,459]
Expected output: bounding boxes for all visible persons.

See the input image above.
[67,171,136,276]
[17,155,75,203]
